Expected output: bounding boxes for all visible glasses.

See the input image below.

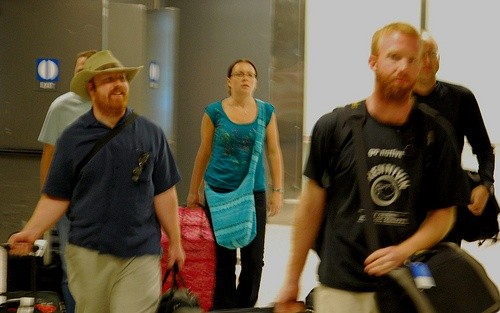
[230,73,256,79]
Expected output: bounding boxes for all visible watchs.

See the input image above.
[481,180,494,197]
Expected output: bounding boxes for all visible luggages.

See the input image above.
[161,202,215,313]
[0,243,72,313]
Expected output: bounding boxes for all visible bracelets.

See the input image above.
[271,187,284,194]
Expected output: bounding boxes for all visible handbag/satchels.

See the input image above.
[156,260,203,313]
[204,172,257,249]
[374,242,500,313]
[454,170,500,246]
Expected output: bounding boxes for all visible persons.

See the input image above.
[272,21,467,313]
[6,50,186,312]
[186,57,285,312]
[411,30,496,247]
[34,49,100,313]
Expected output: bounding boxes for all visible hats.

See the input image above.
[70,49,144,100]
